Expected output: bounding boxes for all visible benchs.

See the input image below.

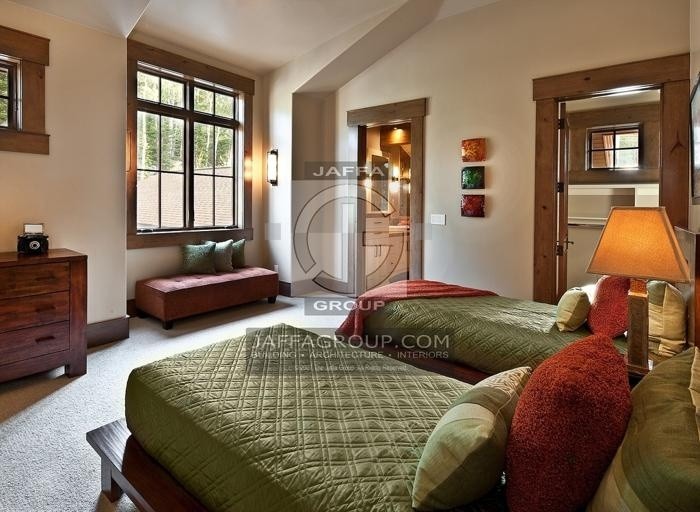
[135,265,279,330]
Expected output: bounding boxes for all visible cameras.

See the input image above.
[17,232,50,254]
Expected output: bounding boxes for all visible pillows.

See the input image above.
[181,239,247,275]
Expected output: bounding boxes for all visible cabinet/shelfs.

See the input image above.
[0,248,88,383]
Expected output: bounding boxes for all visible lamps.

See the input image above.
[585,205,689,380]
[266,149,278,186]
